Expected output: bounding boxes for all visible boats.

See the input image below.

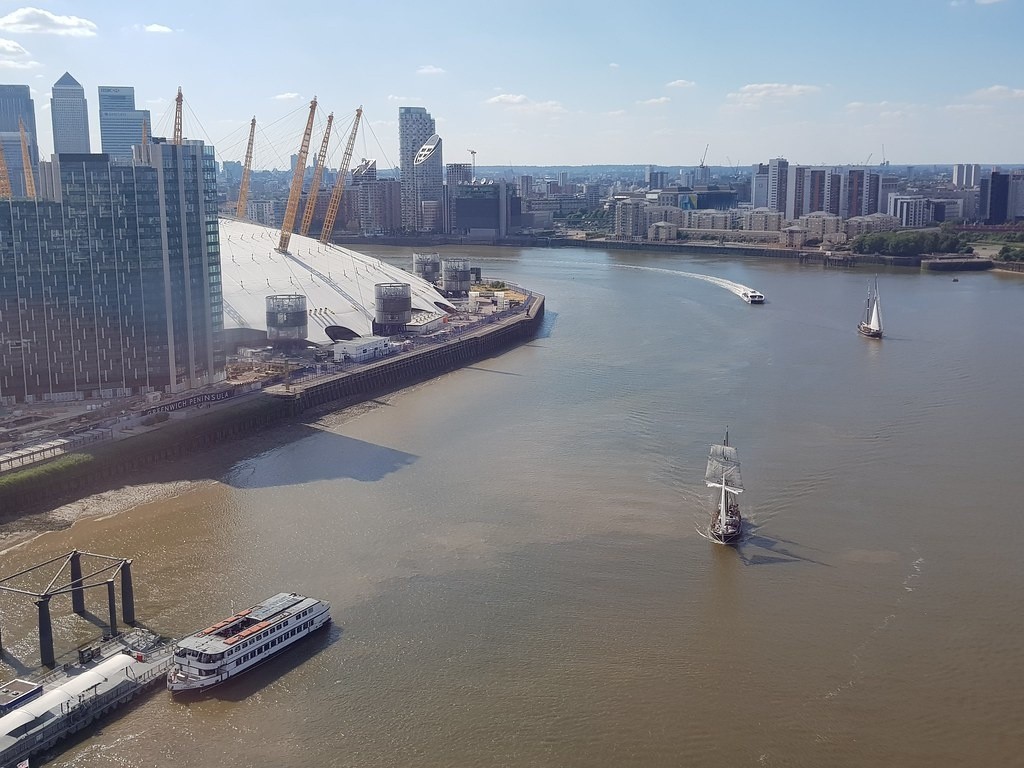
[162,591,333,694]
[741,289,765,305]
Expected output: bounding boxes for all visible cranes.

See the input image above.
[142,85,363,255]
[466,148,477,178]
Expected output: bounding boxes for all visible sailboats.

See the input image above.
[702,425,746,546]
[857,273,887,340]
